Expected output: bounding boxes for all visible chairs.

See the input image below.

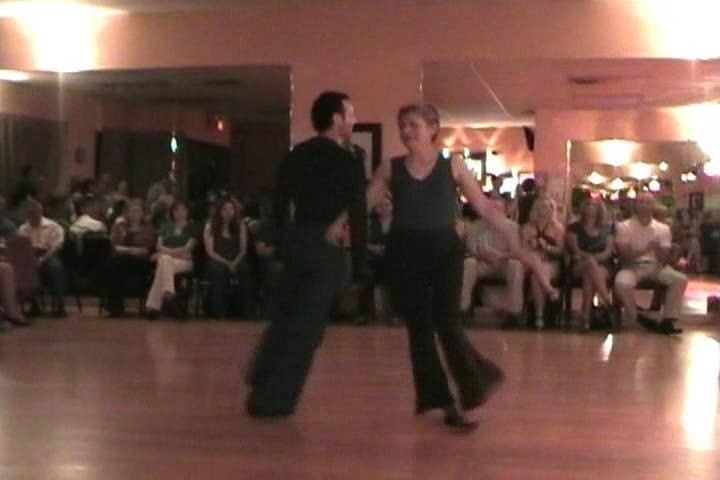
[22,219,681,334]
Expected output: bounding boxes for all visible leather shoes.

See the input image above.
[647,320,683,335]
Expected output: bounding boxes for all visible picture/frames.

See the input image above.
[689,192,703,208]
[348,122,383,180]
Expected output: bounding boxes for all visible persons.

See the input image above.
[245,91,364,417]
[357,194,401,326]
[455,175,718,336]
[324,103,522,434]
[2,175,284,325]
